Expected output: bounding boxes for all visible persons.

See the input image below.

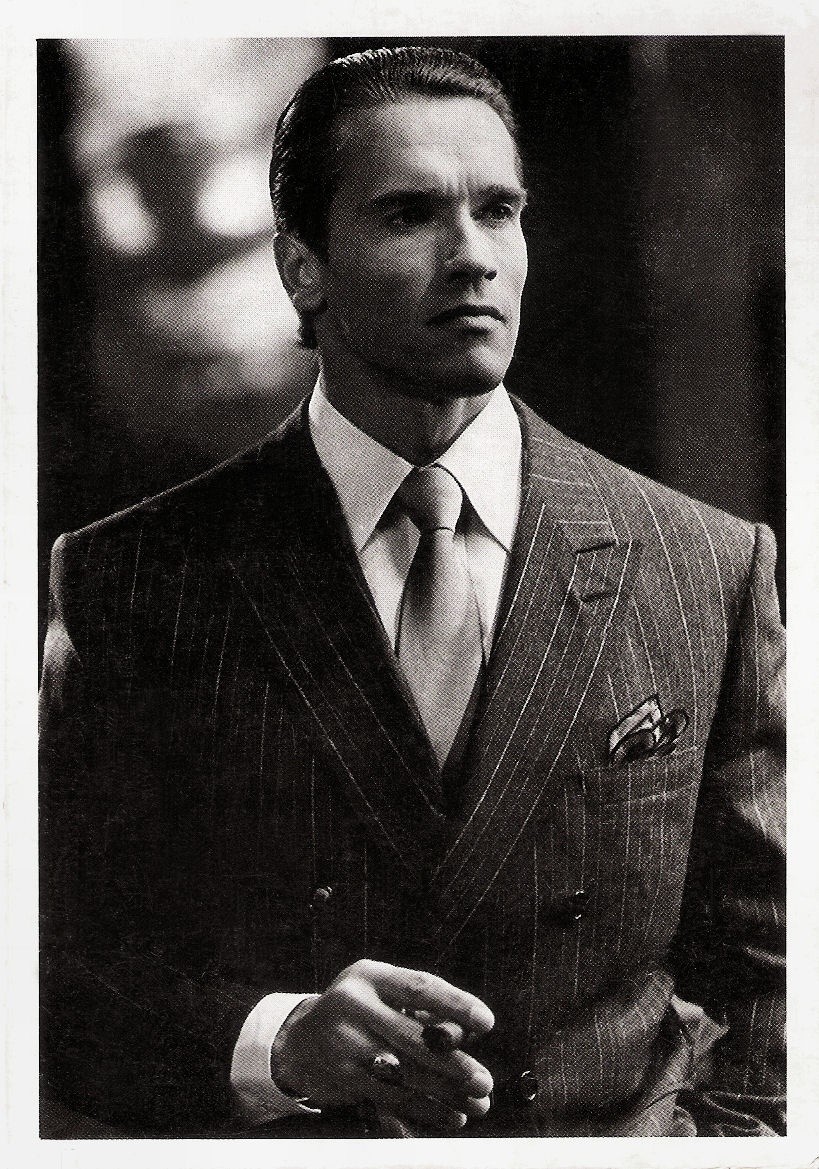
[39,40,787,1137]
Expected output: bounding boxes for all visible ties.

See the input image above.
[392,464,486,803]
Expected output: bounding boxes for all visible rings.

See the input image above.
[371,1053,402,1079]
